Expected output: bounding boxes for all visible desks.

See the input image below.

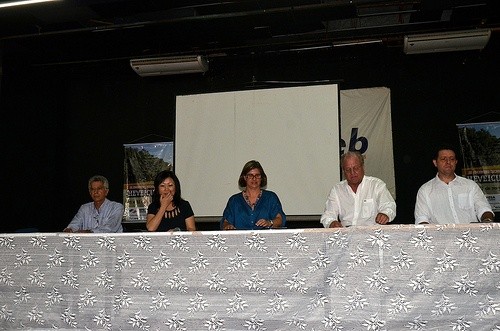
[0,222,500,331]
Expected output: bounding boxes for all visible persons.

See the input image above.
[319,151,397,228]
[219,160,286,230]
[414,143,495,224]
[146,170,196,232]
[63,175,124,232]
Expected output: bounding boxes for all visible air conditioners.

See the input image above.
[402,30,492,55]
[129,54,210,76]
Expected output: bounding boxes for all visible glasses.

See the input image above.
[247,174,262,179]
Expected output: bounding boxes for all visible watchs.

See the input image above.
[268,220,273,227]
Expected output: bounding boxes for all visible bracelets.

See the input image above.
[482,218,493,222]
